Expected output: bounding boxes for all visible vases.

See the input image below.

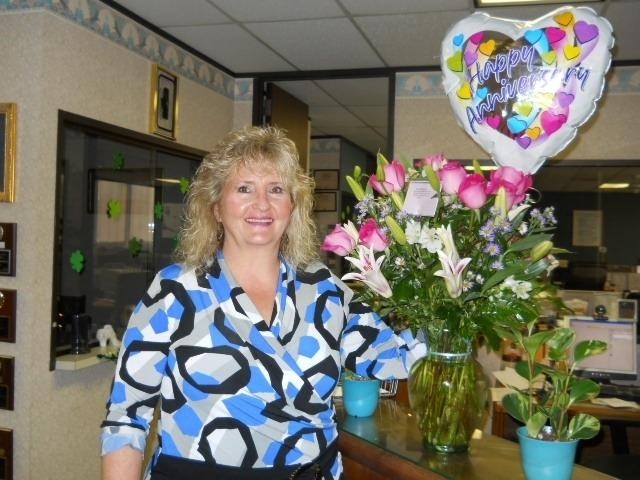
[407,340,491,453]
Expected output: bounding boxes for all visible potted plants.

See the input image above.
[342,370,382,417]
[498,301,607,480]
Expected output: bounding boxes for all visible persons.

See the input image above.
[98,121,429,480]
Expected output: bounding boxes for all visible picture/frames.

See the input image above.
[149,61,180,141]
[1,101,19,202]
[311,168,340,214]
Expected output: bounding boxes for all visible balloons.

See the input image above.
[439,4,616,177]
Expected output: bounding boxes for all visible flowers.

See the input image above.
[319,149,573,444]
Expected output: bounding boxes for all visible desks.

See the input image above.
[488,385,640,463]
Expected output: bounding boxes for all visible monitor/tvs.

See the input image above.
[568,315,637,381]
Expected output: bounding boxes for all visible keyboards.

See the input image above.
[595,383,639,402]
